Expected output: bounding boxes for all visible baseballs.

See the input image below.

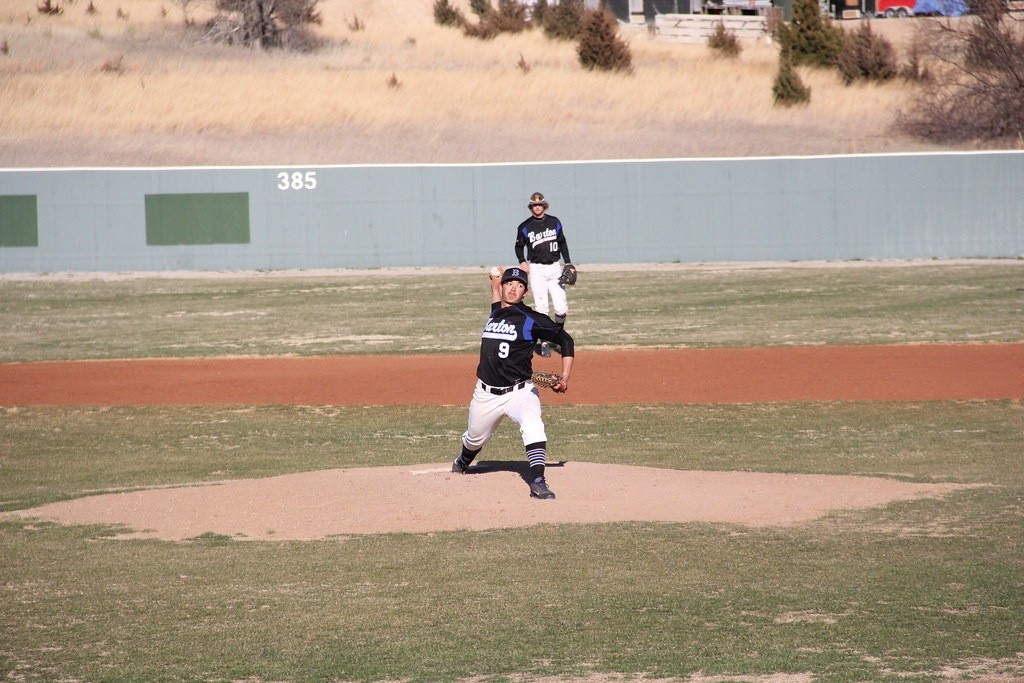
[491,266,500,278]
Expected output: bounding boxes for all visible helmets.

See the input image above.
[528,192,548,210]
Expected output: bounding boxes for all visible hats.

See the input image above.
[500,268,528,284]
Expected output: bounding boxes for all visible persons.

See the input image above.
[451,266,574,499]
[515,192,577,355]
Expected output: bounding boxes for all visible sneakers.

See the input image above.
[541,342,551,358]
[452,454,472,473]
[529,481,556,499]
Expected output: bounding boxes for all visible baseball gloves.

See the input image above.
[530,371,567,392]
[557,264,578,286]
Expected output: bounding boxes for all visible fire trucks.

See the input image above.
[875,0,936,18]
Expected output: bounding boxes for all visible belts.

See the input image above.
[482,382,525,395]
